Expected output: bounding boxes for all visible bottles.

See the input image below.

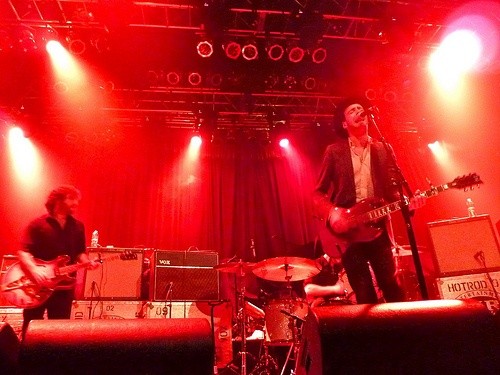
[91,230,98,248]
[465,197,475,217]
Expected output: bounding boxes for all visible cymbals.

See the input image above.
[213,262,256,273]
[252,256,322,282]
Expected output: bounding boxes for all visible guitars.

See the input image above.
[2,250,138,308]
[320,172,481,258]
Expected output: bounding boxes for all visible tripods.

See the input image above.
[220,269,282,375]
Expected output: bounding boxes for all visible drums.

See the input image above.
[197,273,308,347]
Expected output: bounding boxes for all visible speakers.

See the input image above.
[292,298,500,375]
[427,213,500,277]
[0,245,220,375]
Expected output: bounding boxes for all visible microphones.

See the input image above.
[474,251,483,259]
[252,240,256,257]
[91,280,96,290]
[357,106,375,121]
[165,282,173,292]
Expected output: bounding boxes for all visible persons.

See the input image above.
[19,184,103,340]
[309,97,415,304]
[302,253,357,307]
